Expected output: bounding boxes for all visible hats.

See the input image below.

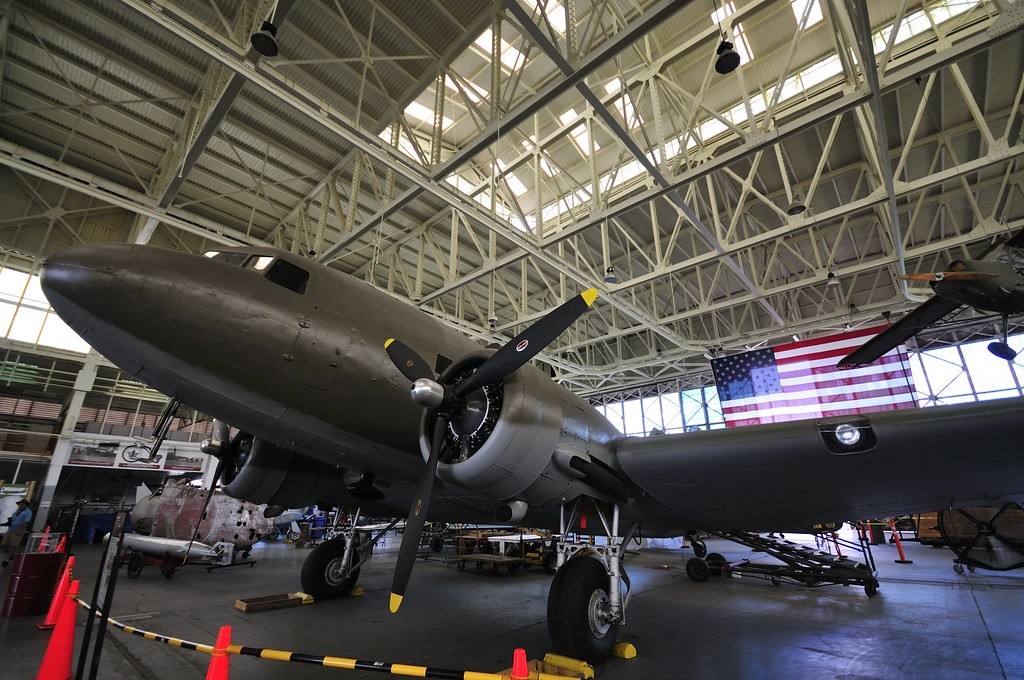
[16,499,30,507]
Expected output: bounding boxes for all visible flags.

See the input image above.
[710,322,921,428]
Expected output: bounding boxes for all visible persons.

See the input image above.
[1,498,32,567]
[315,512,326,540]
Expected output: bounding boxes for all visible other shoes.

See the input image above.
[2,561,9,566]
[10,555,15,560]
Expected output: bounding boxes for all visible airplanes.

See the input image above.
[836,227,1024,371]
[37,245,1024,666]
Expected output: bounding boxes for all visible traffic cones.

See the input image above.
[33,580,82,680]
[205,625,232,680]
[36,526,51,552]
[35,555,76,629]
[55,535,67,553]
[510,648,530,680]
